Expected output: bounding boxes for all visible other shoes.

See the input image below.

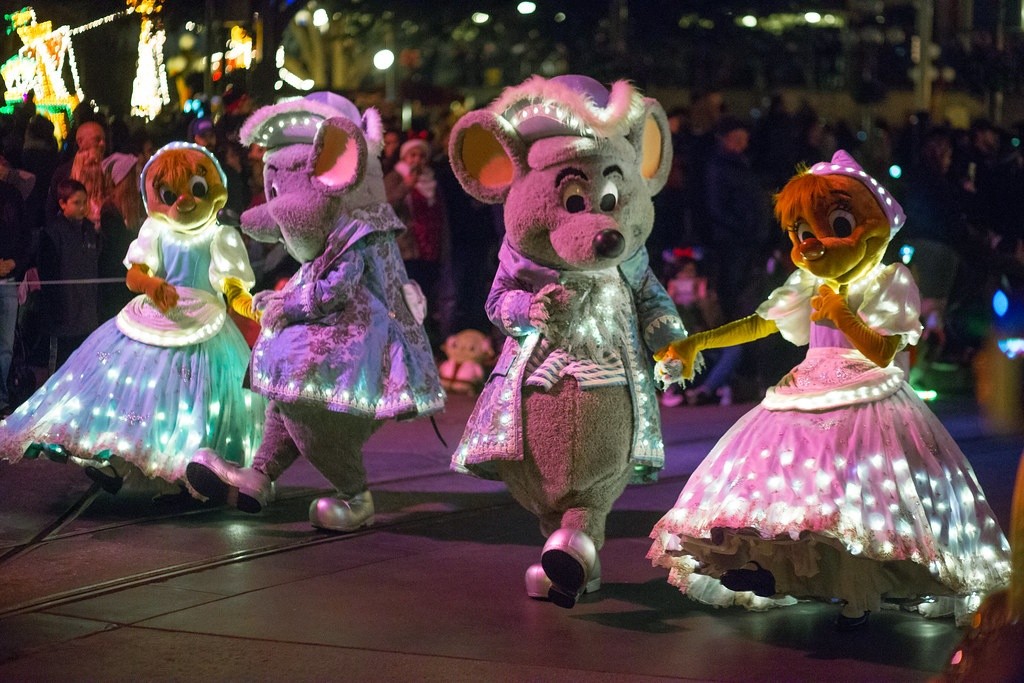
[0,406,14,418]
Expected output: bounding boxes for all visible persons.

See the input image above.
[0,87,1023,416]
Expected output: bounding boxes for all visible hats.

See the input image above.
[101,152,138,186]
[399,129,430,164]
[191,119,215,135]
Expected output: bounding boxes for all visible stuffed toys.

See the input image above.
[185,91,448,535]
[645,152,1014,630]
[0,144,269,511]
[450,75,705,607]
